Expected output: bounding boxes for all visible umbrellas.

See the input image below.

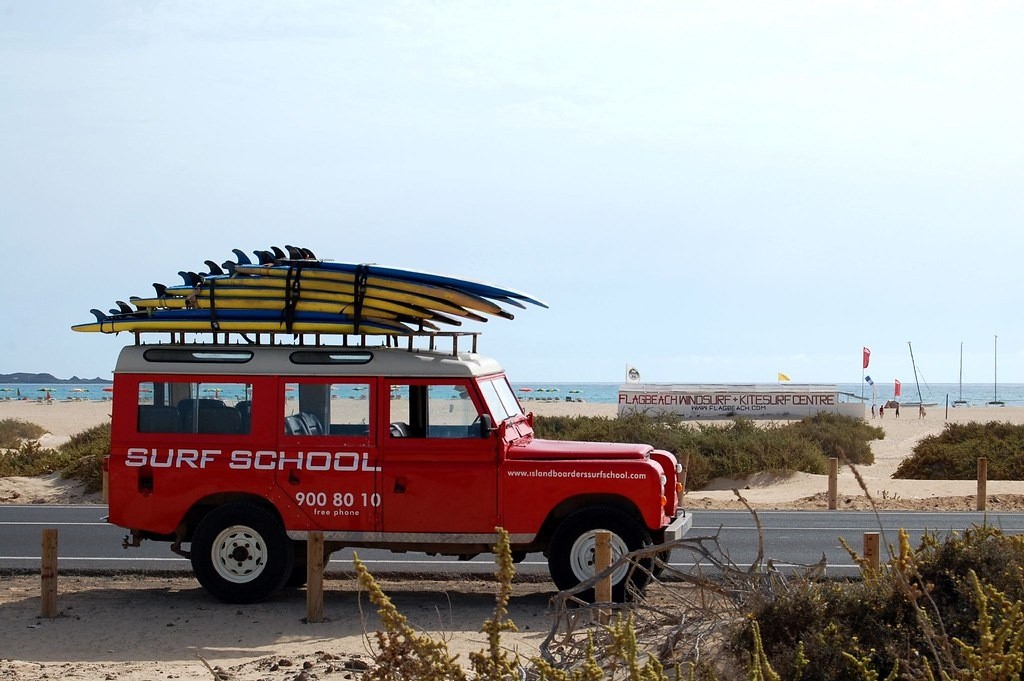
[215,388,219,399]
[203,388,223,397]
[391,385,404,396]
[71,388,88,396]
[550,388,559,397]
[285,387,295,391]
[331,386,339,395]
[352,386,364,391]
[535,388,549,400]
[0,388,15,396]
[38,387,56,399]
[139,388,150,396]
[17,388,21,396]
[569,390,579,398]
[102,387,113,397]
[518,388,532,397]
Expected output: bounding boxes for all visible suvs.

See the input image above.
[99,338,693,609]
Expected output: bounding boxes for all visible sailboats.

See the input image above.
[899,340,939,407]
[949,341,977,408]
[984,334,1007,407]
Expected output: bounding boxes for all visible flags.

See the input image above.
[864,348,871,368]
[778,372,790,382]
[865,376,873,385]
[895,380,901,396]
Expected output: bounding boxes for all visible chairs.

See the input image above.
[519,396,584,403]
[138,399,251,434]
[285,412,323,436]
[0,396,111,404]
[389,422,409,438]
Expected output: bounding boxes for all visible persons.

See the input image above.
[895,402,900,418]
[918,403,925,419]
[872,403,876,419]
[879,404,884,419]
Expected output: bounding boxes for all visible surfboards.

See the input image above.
[70,244,549,336]
